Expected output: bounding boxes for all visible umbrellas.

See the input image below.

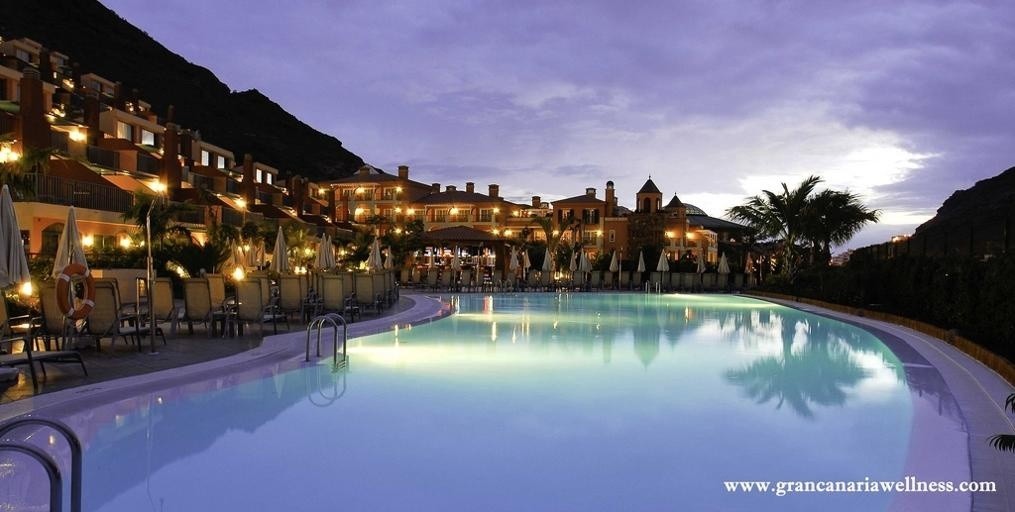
[223,235,267,271]
[451,245,462,285]
[313,232,329,269]
[655,247,670,290]
[717,251,732,274]
[48,204,89,351]
[383,244,394,271]
[326,233,336,270]
[579,247,591,283]
[697,250,707,285]
[508,244,519,270]
[366,236,383,271]
[585,252,593,271]
[636,250,646,290]
[269,224,292,273]
[426,248,435,269]
[524,250,531,269]
[609,250,619,289]
[0,184,33,291]
[542,247,554,283]
[569,248,578,290]
[743,252,755,275]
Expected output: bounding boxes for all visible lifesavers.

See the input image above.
[57,263,96,320]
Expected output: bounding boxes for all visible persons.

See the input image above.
[581,271,592,292]
